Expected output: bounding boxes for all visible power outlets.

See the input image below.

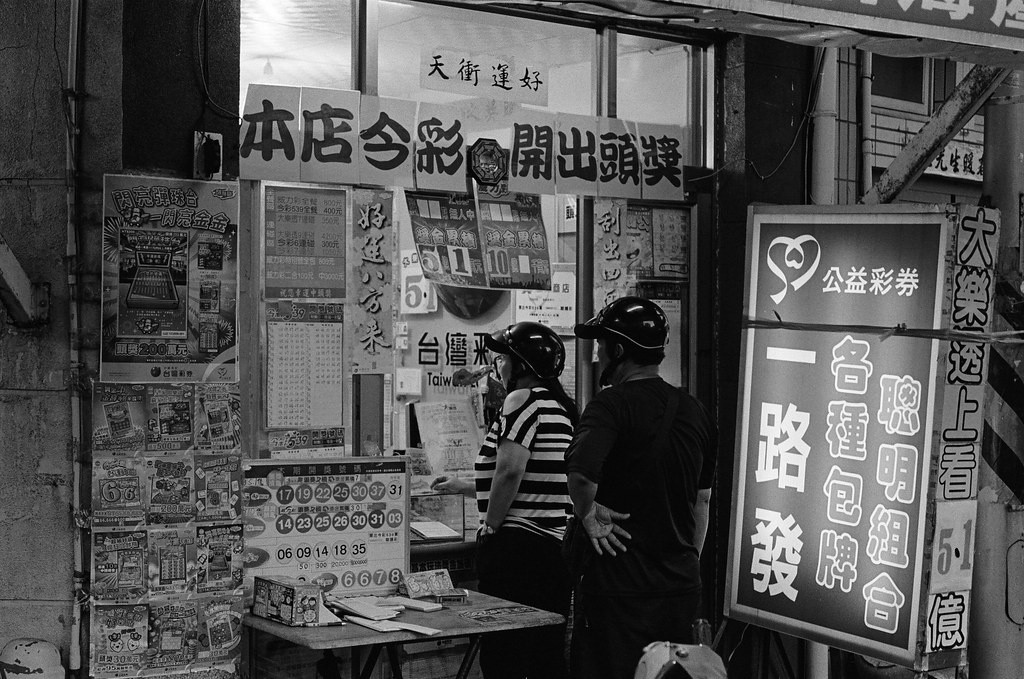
[193,131,223,182]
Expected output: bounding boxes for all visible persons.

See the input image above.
[564,296,718,679]
[430,322,579,679]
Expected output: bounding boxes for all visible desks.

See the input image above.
[241,588,565,679]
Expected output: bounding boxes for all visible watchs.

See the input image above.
[483,521,495,534]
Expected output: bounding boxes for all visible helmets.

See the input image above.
[484,321,566,381]
[573,295,670,349]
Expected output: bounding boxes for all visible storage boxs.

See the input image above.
[410,487,465,543]
[252,575,320,626]
